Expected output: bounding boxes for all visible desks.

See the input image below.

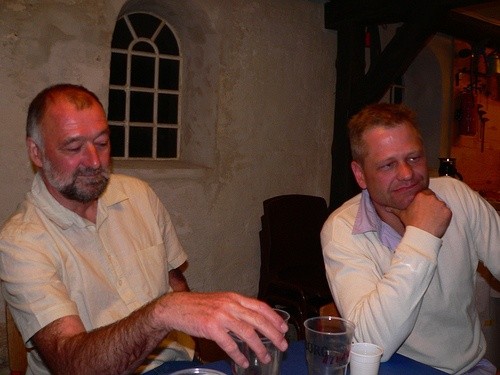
[166,339,457,375]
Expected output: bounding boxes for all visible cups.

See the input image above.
[349,343,383,375]
[169,368,226,375]
[230,309,290,375]
[303,316,355,375]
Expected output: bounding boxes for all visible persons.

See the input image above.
[321,102,500,375]
[1,84,288,375]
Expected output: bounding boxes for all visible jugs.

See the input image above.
[438,157,463,181]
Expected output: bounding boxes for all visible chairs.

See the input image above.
[258,194,332,341]
